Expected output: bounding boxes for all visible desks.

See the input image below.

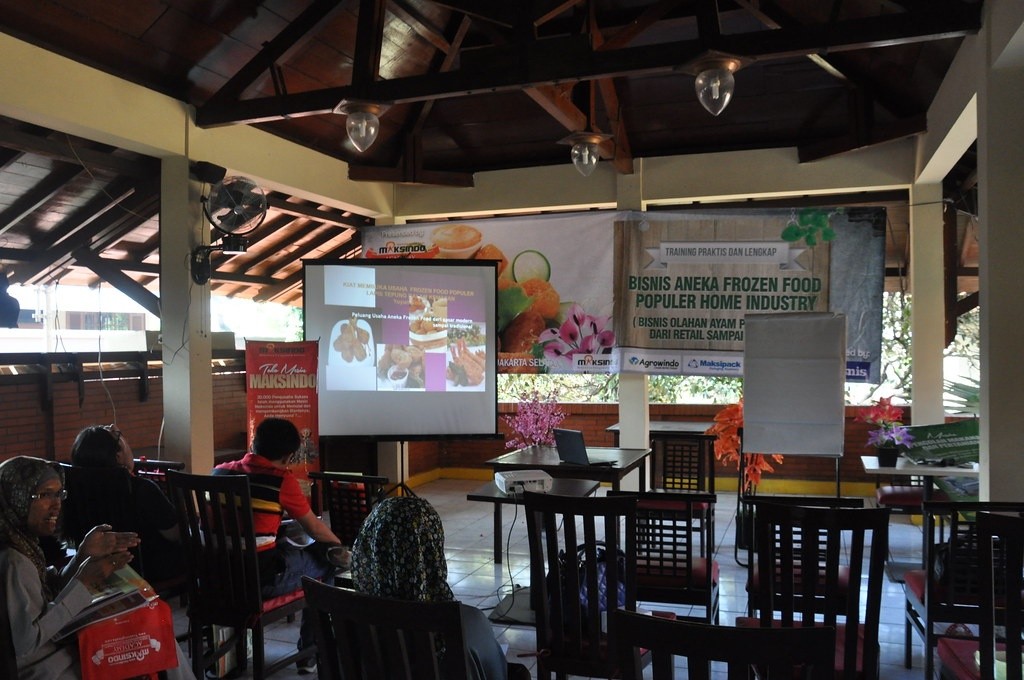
[861,455,981,581]
[606,418,717,444]
[463,477,603,566]
[488,440,651,495]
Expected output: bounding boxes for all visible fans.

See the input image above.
[190,165,269,285]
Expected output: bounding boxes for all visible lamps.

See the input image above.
[557,127,614,177]
[324,92,394,154]
[676,47,754,115]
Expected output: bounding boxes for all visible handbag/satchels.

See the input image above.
[78,596,179,680]
[546,540,627,611]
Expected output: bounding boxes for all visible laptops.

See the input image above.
[553,428,617,465]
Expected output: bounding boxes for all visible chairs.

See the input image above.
[44,458,460,680]
[524,431,1024,680]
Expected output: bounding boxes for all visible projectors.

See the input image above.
[495,469,553,494]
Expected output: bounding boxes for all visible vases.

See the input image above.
[873,443,904,468]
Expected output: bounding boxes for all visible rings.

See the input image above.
[112,561,116,566]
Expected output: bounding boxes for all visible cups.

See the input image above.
[387,365,409,388]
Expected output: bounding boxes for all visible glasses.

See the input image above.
[102,425,124,444]
[31,490,68,502]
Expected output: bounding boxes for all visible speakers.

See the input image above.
[194,161,228,183]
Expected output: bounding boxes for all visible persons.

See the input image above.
[62,421,203,581]
[199,416,352,680]
[346,494,508,680]
[0,455,143,680]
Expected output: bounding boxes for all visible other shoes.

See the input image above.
[295,643,317,674]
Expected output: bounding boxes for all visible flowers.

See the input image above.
[866,421,917,449]
[537,303,614,372]
[499,379,568,451]
